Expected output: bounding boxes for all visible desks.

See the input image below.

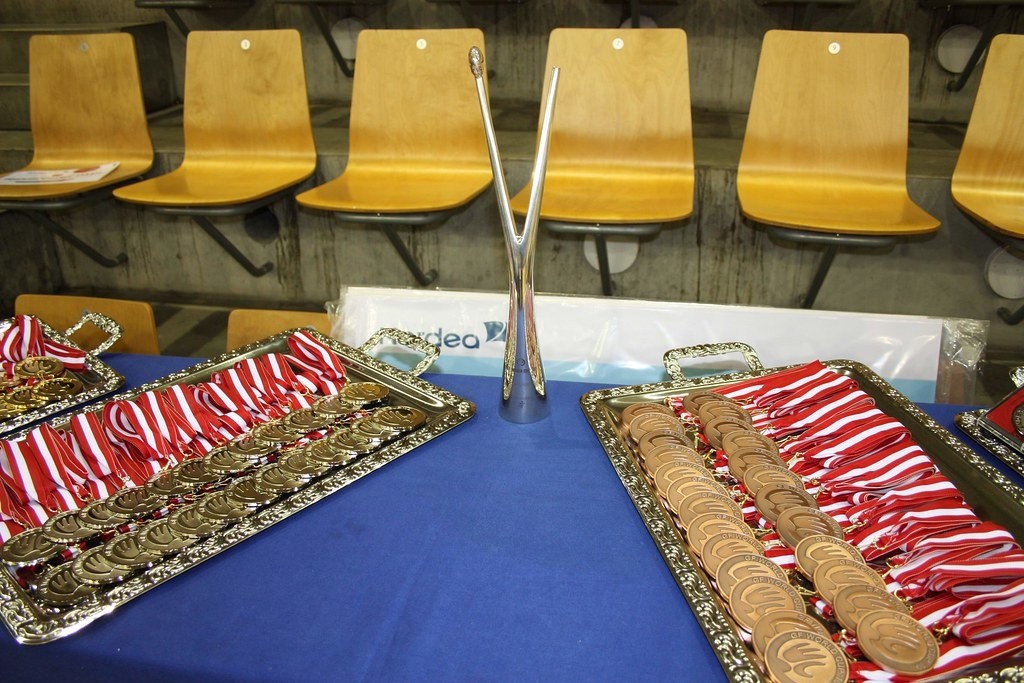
[0,356,1024,683]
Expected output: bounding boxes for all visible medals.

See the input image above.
[0,356,428,605]
[622,393,940,683]
[1013,402,1024,436]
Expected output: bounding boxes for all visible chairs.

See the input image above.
[0,32,155,269]
[226,309,342,352]
[295,27,493,288]
[949,33,1024,326]
[112,29,317,278]
[15,294,160,356]
[919,0,1024,93]
[511,27,696,296]
[736,30,943,310]
[135,0,254,38]
